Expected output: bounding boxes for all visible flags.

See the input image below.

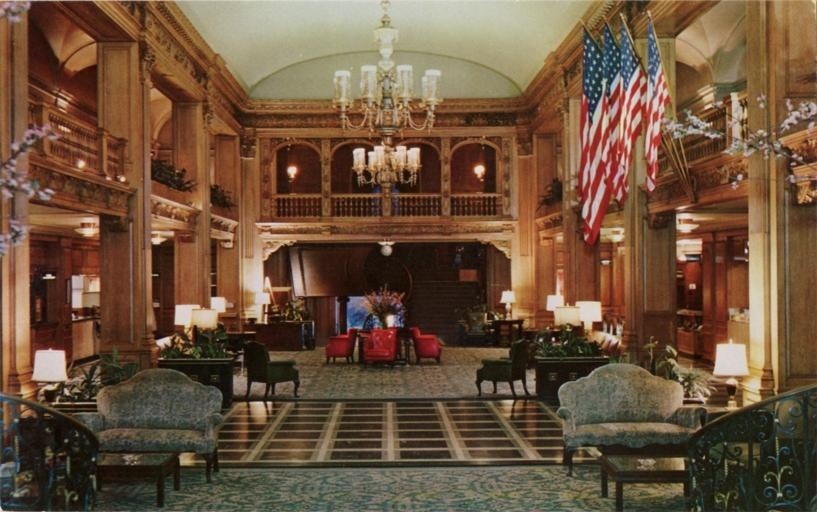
[576,19,670,246]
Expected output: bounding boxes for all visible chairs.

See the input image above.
[326,328,442,365]
[493,319,524,345]
[682,383,816,511]
[459,311,493,346]
[0,394,102,512]
[244,342,300,402]
[475,339,530,399]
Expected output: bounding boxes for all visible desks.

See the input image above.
[700,404,748,430]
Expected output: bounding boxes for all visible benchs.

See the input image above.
[556,364,706,476]
[84,368,224,483]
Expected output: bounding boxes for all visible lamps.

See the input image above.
[378,240,394,256]
[73,218,99,239]
[174,297,228,332]
[151,233,167,247]
[604,228,625,244]
[500,290,516,321]
[676,219,701,235]
[545,295,603,334]
[712,341,751,411]
[255,291,271,321]
[330,1,441,184]
[31,346,68,403]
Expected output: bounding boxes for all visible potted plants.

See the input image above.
[156,329,236,412]
[50,348,141,413]
[640,334,712,408]
[533,328,610,401]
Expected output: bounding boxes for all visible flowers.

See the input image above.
[656,95,817,186]
[1,2,61,256]
[363,285,406,328]
